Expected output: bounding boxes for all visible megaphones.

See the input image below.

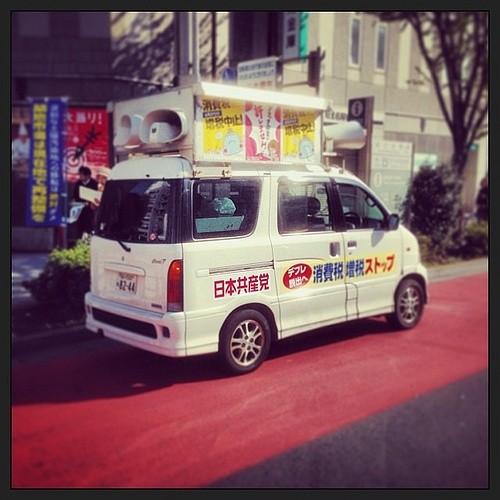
[137,107,188,145]
[112,113,143,149]
[322,118,368,154]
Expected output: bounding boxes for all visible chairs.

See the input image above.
[284,194,326,231]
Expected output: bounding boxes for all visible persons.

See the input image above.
[71,167,99,239]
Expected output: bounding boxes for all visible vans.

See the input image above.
[81,79,429,374]
